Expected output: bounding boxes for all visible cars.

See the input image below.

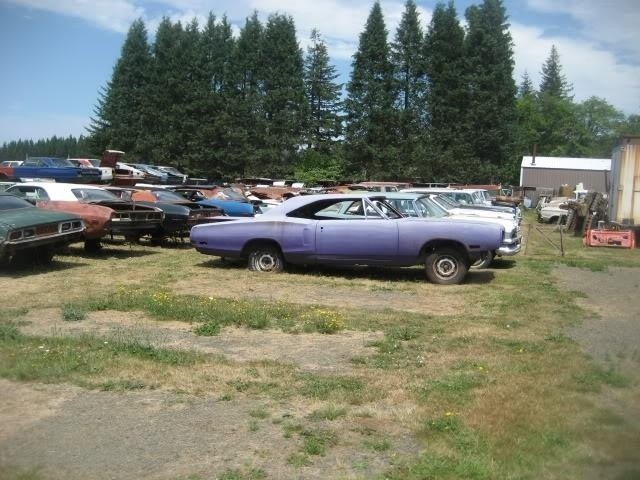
[539,190,590,224]
